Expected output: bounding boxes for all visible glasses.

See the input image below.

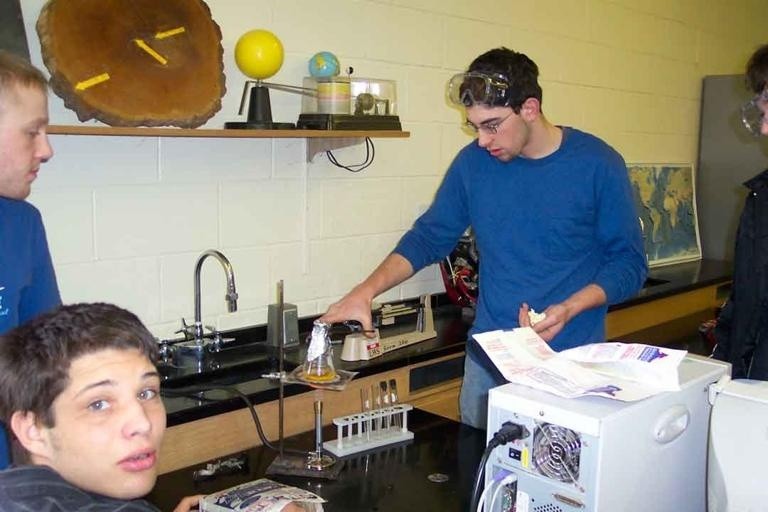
[462,111,517,138]
[446,70,506,108]
[738,90,768,139]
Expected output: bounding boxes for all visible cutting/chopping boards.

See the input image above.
[35,0,228,129]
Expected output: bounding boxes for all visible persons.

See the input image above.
[713,41,767,383]
[0,300,313,512]
[0,49,66,477]
[313,44,651,511]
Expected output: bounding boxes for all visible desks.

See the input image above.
[145,404,487,512]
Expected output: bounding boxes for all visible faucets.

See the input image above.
[194,249,238,346]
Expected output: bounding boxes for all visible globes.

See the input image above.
[308,51,340,78]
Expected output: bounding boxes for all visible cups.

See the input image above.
[340,335,361,362]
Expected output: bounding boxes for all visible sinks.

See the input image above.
[160,356,303,397]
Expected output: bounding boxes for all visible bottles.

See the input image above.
[302,320,337,382]
[358,378,403,439]
